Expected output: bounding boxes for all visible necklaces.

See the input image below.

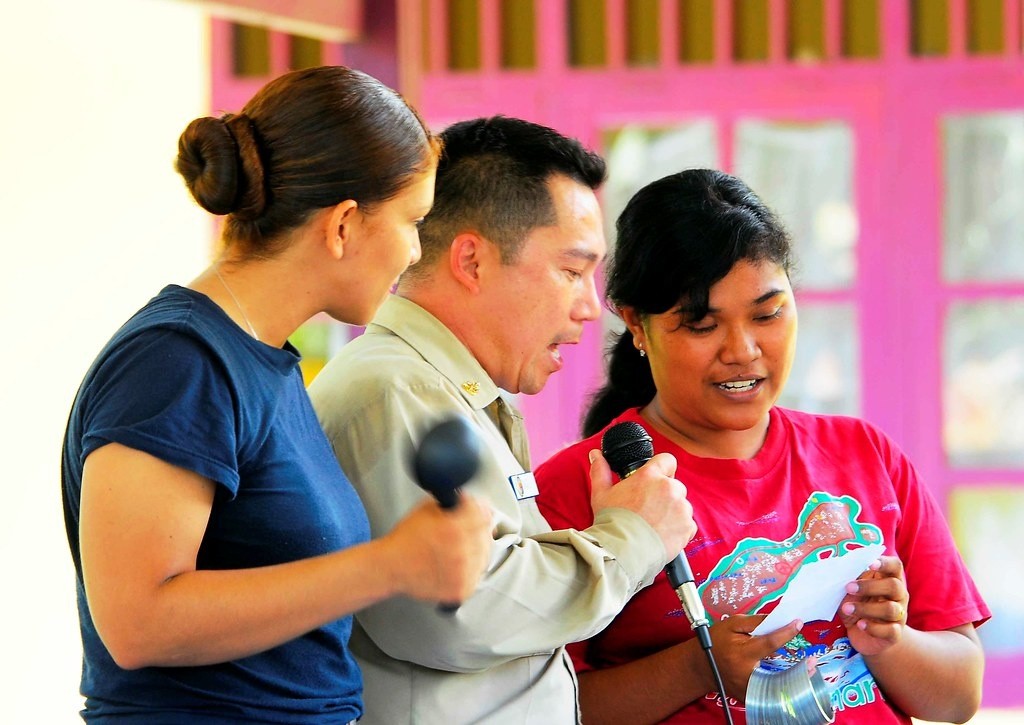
[204,259,260,342]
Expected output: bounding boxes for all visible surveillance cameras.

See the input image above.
[602,420,715,649]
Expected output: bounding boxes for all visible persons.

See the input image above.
[61,65,494,725]
[305,116,697,724]
[535,170,992,724]
